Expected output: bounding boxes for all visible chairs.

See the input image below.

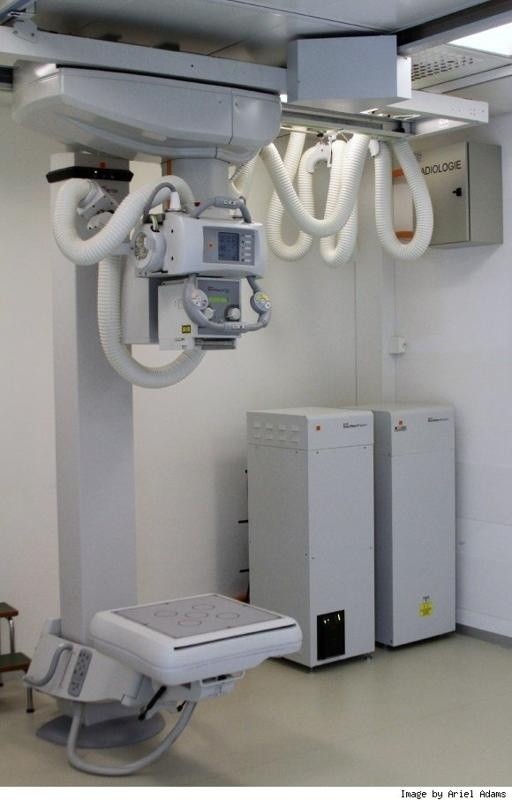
[0,602,34,712]
